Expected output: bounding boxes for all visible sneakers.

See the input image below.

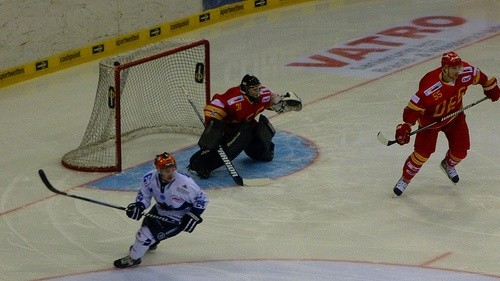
[129,243,158,251]
[440,159,460,184]
[392,177,409,197]
[113,256,142,269]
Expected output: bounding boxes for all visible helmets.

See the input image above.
[441,52,461,66]
[241,74,263,99]
[155,152,176,170]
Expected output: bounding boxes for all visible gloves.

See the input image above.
[482,77,500,101]
[394,123,411,146]
[124,202,146,221]
[179,211,203,233]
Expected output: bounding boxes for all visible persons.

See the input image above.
[393,52,500,196]
[113,152,208,268]
[188,75,302,179]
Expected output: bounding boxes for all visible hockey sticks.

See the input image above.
[180,84,270,185]
[38,169,181,225]
[377,97,489,147]
